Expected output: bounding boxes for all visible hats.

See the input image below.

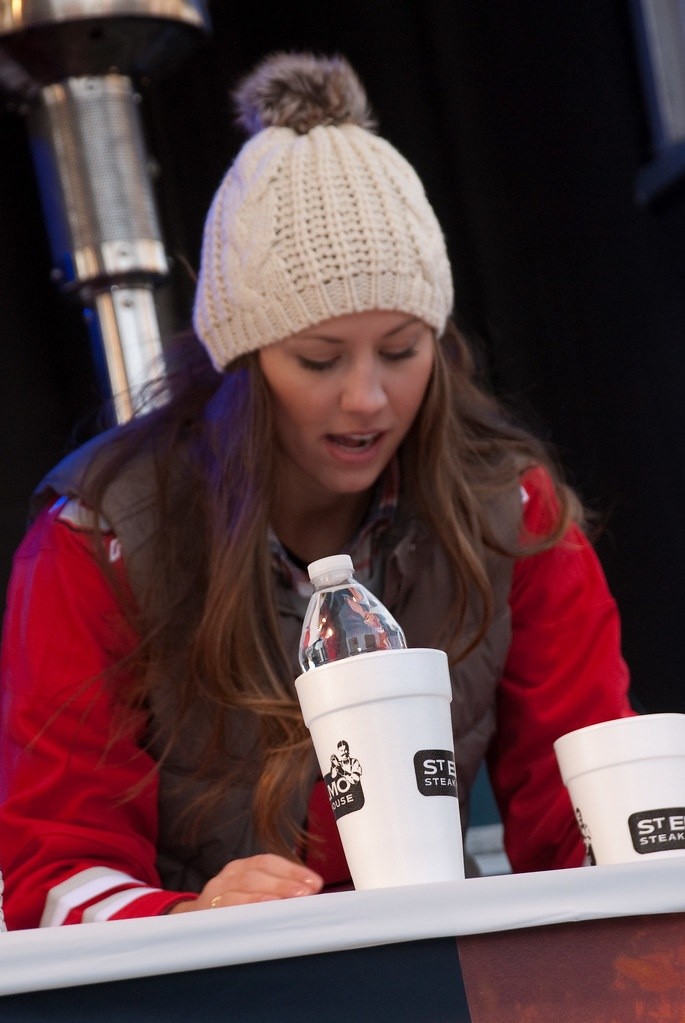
[192,52,456,370]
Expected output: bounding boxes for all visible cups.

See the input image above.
[552,713,685,867]
[294,648,464,891]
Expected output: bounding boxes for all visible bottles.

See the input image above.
[297,554,408,674]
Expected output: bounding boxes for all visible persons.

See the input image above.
[0,59,641,932]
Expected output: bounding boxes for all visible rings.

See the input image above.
[210,895,222,908]
